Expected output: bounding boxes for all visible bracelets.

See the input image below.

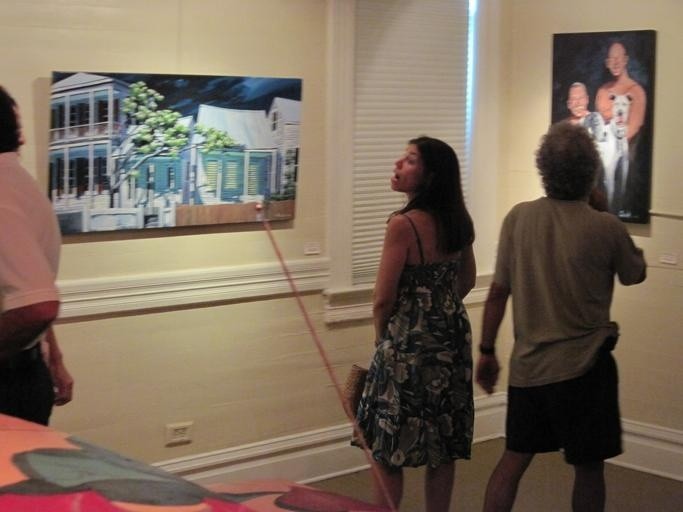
[479,345,495,354]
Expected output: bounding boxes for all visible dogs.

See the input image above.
[592,94,634,207]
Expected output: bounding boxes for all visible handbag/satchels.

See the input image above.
[341,363,368,417]
[604,336,617,350]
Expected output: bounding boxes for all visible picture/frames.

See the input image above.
[547,29,656,223]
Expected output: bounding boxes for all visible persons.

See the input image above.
[562,83,593,125]
[349,136,477,512]
[0,84,73,426]
[474,120,646,512]
[594,42,648,219]
